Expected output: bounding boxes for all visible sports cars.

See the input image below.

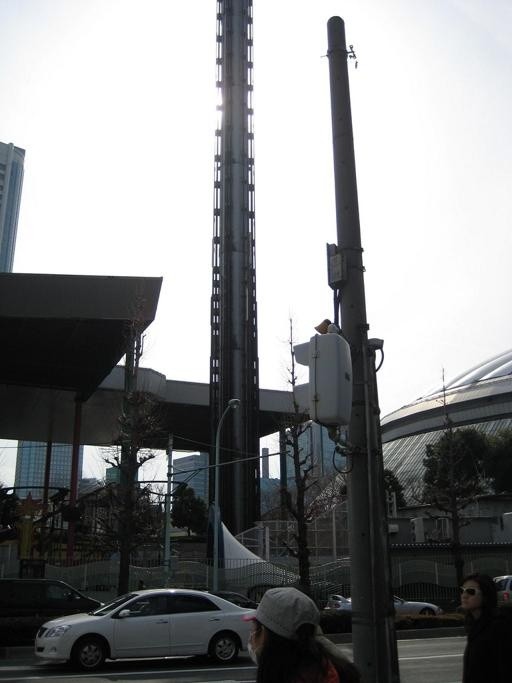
[328,592,442,617]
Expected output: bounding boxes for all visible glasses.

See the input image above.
[457,586,477,596]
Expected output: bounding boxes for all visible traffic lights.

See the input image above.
[293,332,351,426]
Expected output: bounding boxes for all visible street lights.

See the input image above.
[212,397,239,590]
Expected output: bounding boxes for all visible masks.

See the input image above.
[245,627,267,666]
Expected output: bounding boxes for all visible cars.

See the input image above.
[205,591,258,610]
[33,588,260,673]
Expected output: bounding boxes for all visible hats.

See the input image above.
[239,585,324,644]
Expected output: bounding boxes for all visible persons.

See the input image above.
[243,586,364,682]
[458,572,511,682]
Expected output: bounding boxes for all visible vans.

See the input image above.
[0,577,105,650]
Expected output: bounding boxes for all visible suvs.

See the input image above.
[492,573,512,608]
